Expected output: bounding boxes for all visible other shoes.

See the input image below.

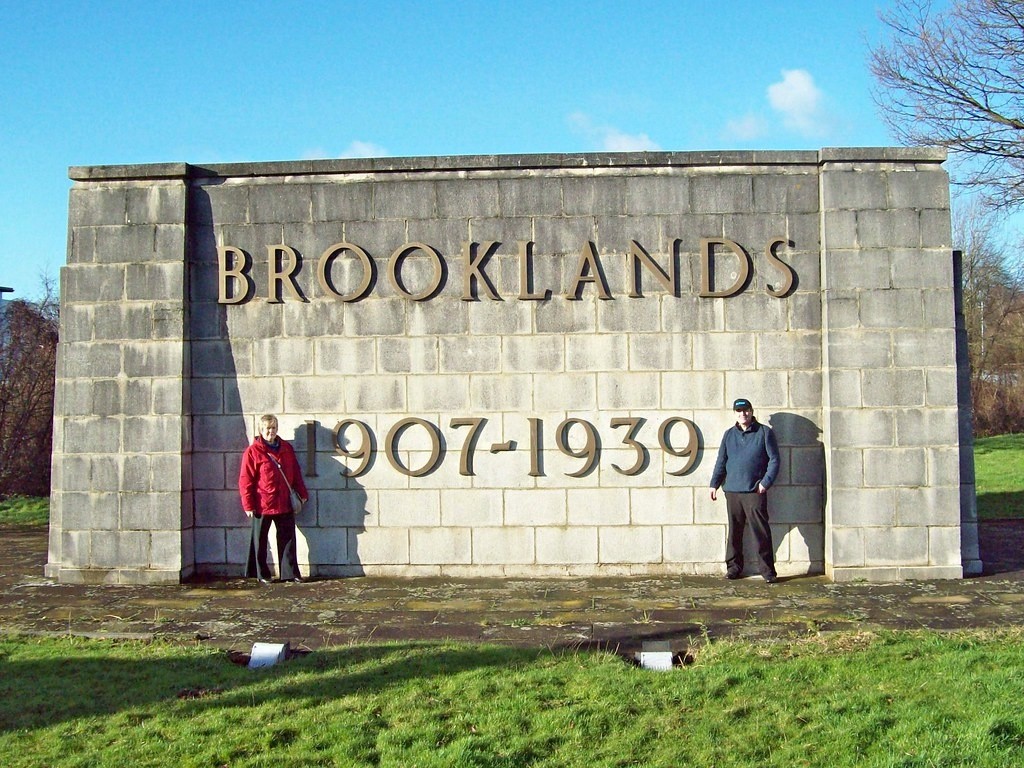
[766,576,776,583]
[724,573,736,578]
[294,577,304,582]
[260,578,273,583]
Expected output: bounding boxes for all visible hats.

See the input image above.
[732,399,751,409]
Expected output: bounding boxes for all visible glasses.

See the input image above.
[735,409,749,412]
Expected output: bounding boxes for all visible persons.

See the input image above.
[710,399,780,583]
[239,414,310,583]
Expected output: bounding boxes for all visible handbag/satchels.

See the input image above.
[289,489,304,516]
